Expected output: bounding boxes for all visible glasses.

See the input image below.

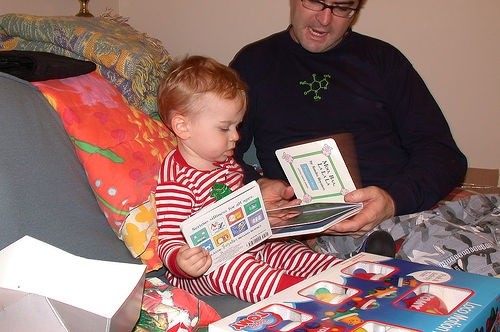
[300,0,360,18]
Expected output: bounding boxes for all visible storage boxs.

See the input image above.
[0,235,148,332]
[208,252,500,332]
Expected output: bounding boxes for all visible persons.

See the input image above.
[157,55,397,302]
[226,1,469,256]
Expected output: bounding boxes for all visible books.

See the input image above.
[265,137,363,238]
[180,179,273,277]
